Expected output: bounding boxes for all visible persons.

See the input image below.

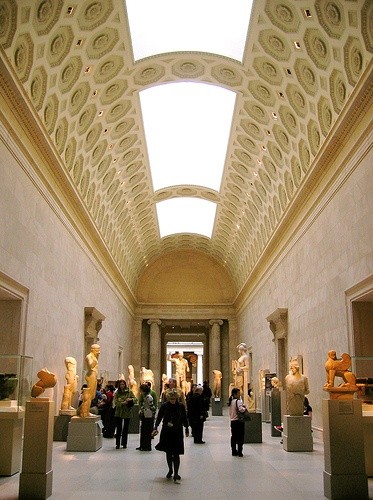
[77,376,167,452]
[227,388,247,457]
[151,388,190,482]
[161,378,188,421]
[235,343,255,408]
[248,382,255,411]
[189,385,207,444]
[161,374,169,395]
[273,396,313,444]
[201,380,212,419]
[61,357,79,411]
[186,383,197,437]
[265,377,280,397]
[139,367,155,390]
[167,349,190,395]
[127,365,137,398]
[212,370,223,398]
[285,361,310,416]
[78,344,101,418]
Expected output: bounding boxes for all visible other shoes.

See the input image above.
[173,473,180,482]
[165,469,173,478]
[115,445,119,449]
[231,450,238,456]
[122,445,126,448]
[274,426,283,432]
[238,451,243,457]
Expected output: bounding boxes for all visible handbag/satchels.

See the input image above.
[235,399,251,422]
